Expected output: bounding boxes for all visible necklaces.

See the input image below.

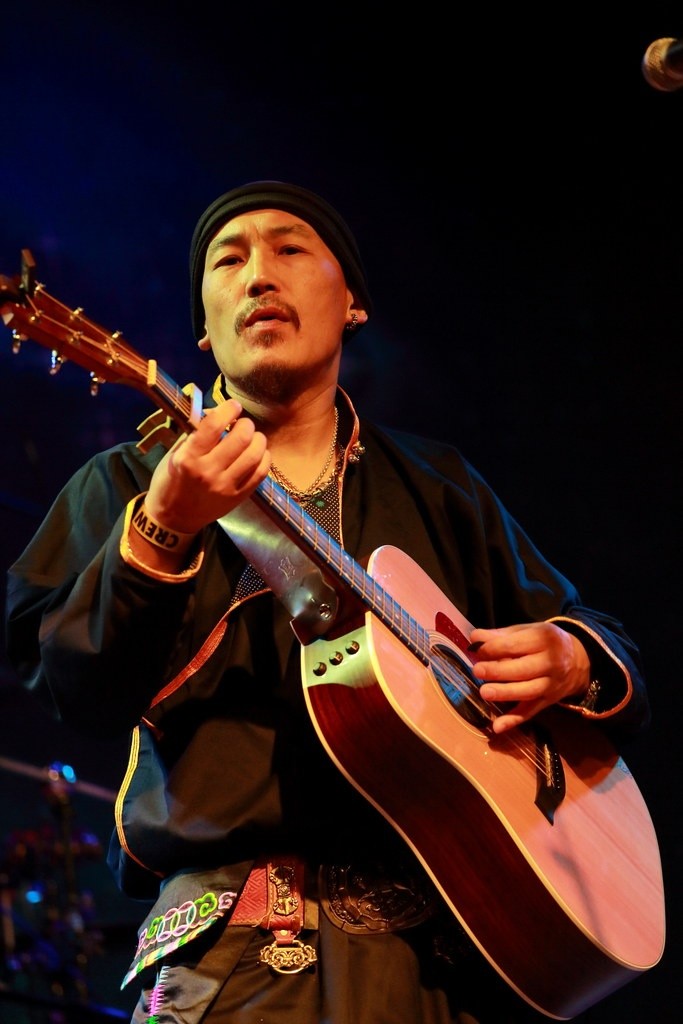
[270,408,345,502]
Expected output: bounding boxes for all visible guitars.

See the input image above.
[0,250,668,1022]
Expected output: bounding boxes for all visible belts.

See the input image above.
[224,853,448,935]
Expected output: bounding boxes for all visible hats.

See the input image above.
[186,179,375,331]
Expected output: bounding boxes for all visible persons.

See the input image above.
[0,180,642,1024]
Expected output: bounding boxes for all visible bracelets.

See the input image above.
[579,680,601,708]
[133,510,194,552]
